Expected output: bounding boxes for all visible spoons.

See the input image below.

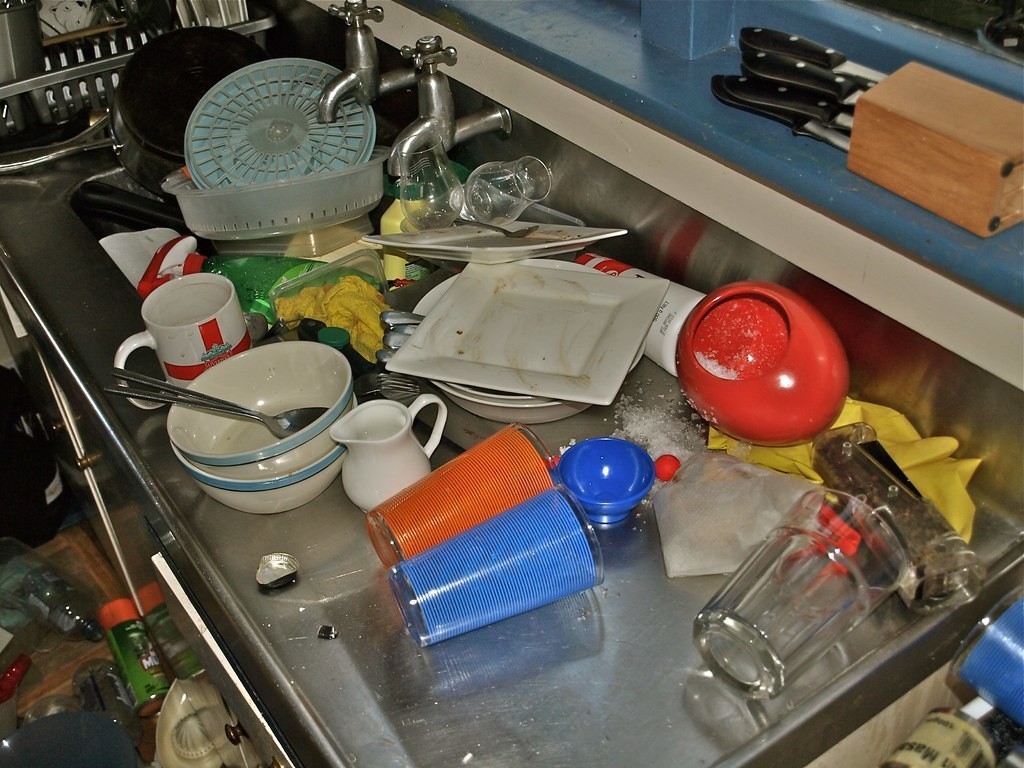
[104,383,341,443]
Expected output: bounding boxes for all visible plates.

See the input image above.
[357,220,671,422]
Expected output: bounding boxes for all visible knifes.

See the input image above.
[710,20,889,153]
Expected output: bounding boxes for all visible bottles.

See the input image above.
[875,706,1002,768]
[0,540,154,768]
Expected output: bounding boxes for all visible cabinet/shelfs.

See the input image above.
[0,297,304,768]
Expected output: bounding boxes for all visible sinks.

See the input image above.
[61,157,226,320]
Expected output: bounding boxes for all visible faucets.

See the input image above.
[303,0,388,125]
[388,34,459,181]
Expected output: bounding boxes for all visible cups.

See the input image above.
[393,134,464,233]
[570,252,707,378]
[694,489,907,698]
[366,419,570,577]
[946,580,1024,755]
[328,393,450,512]
[387,485,606,648]
[449,154,552,225]
[114,273,254,411]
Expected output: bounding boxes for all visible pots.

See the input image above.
[0,25,270,192]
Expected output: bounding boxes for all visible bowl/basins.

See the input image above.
[558,436,657,525]
[674,281,851,448]
[169,393,363,515]
[167,341,356,480]
